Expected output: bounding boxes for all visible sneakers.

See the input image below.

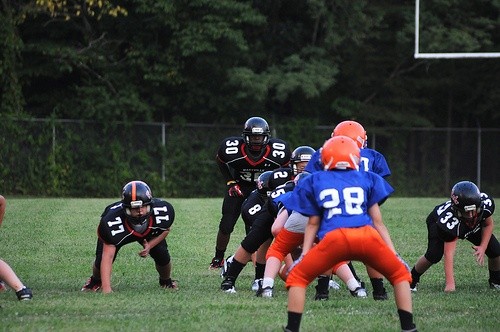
[18,288,31,301]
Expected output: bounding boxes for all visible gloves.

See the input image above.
[227,183,244,199]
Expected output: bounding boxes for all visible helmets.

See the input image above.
[289,145,317,170]
[321,136,360,172]
[450,181,481,223]
[121,181,155,225]
[243,116,271,154]
[330,120,368,149]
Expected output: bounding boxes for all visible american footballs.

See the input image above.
[278,264,288,283]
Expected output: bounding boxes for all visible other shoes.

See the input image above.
[220,255,234,278]
[400,323,418,332]
[349,282,368,300]
[251,278,263,291]
[81,275,102,293]
[158,277,179,291]
[208,257,225,271]
[371,287,389,301]
[314,285,330,301]
[255,280,274,299]
[410,275,419,293]
[488,278,500,289]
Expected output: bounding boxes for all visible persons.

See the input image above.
[81,181,178,293]
[410,180,500,292]
[209,117,293,270]
[283,136,418,332]
[0,195,33,301]
[220,120,391,300]
